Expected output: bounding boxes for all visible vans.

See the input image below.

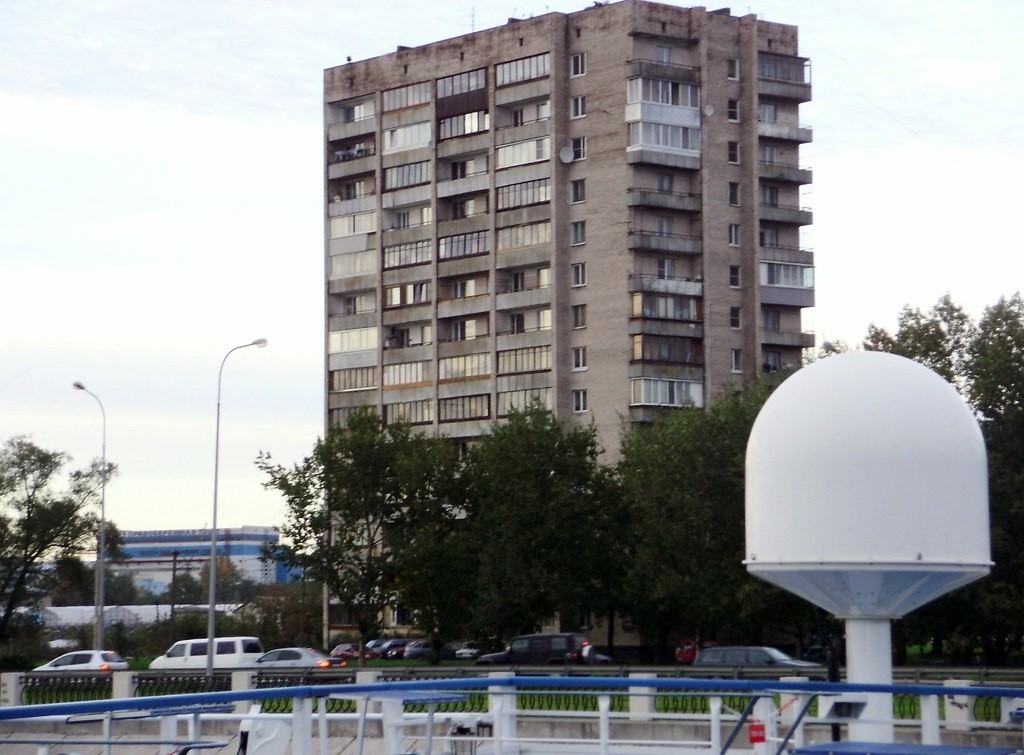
[146,636,265,671]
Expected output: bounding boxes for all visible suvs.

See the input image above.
[473,631,597,668]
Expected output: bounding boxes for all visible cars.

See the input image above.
[331,643,383,660]
[366,638,435,660]
[27,650,131,671]
[698,646,826,682]
[595,652,611,665]
[453,640,477,659]
[249,647,349,669]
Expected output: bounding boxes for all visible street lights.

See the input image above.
[205,338,270,675]
[73,380,106,649]
